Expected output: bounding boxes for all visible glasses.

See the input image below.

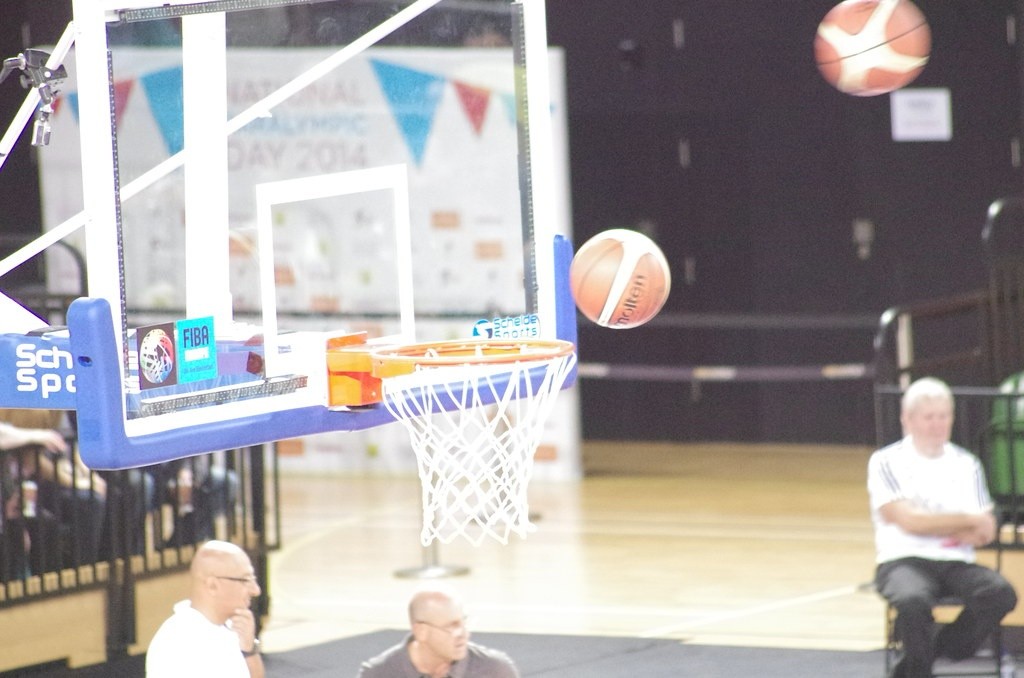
[216,574,257,586]
[417,620,472,637]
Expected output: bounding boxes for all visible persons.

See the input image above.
[144,539,266,677]
[358,590,521,677]
[0,405,250,577]
[865,375,1017,678]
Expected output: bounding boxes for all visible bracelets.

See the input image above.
[241,639,261,657]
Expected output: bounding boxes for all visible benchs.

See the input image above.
[0,403,253,588]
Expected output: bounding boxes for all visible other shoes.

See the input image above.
[892,622,946,678]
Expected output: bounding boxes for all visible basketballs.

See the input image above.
[565,229,672,332]
[811,0,933,98]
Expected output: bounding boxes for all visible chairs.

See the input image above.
[886,595,1004,678]
